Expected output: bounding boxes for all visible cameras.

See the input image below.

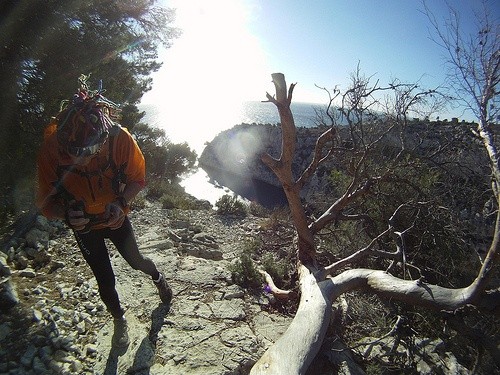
[75,200,110,235]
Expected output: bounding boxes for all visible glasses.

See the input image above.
[66,144,102,158]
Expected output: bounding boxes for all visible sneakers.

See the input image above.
[153,273,172,304]
[114,317,129,346]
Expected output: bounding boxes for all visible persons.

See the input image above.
[35,74,173,348]
